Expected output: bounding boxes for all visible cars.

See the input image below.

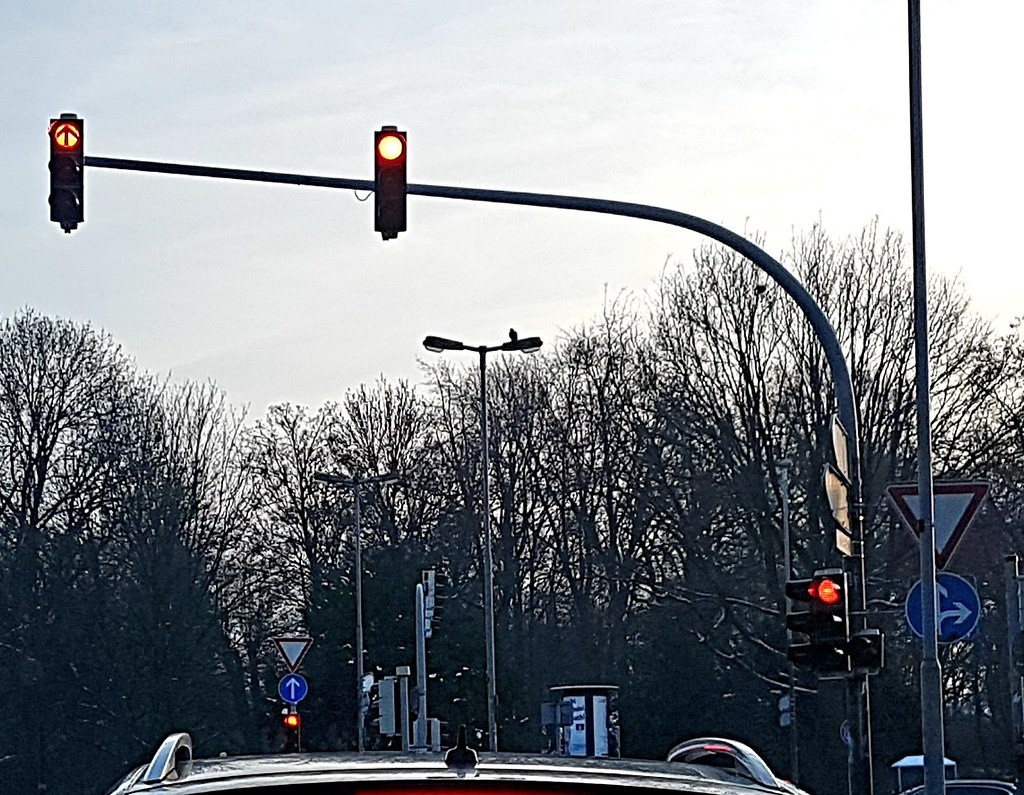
[897,779,1018,795]
[109,731,810,795]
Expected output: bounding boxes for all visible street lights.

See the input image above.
[422,327,546,755]
[311,470,403,750]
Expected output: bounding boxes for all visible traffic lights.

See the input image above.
[423,570,449,638]
[430,718,449,752]
[369,679,395,734]
[45,112,88,234]
[809,569,855,680]
[371,125,409,242]
[786,576,812,665]
[283,713,301,752]
[858,633,885,672]
